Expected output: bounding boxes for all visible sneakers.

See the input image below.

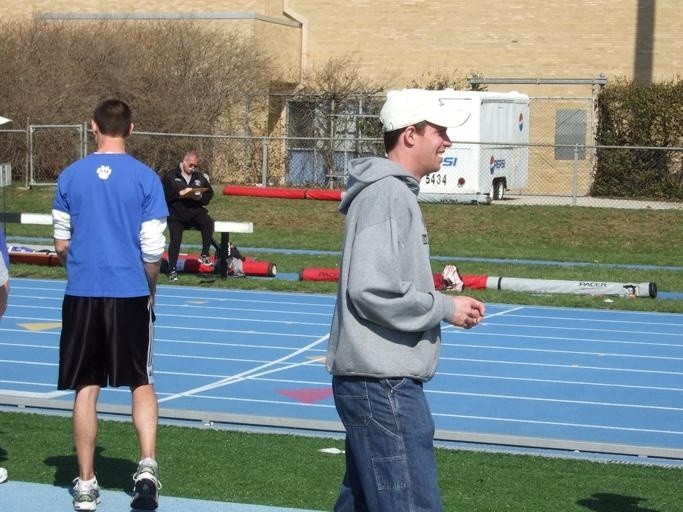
[167,266,179,281]
[72,480,103,511]
[196,254,213,268]
[129,457,163,511]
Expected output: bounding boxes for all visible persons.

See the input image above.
[48,97,171,512]
[323,86,484,511]
[159,150,214,285]
[0,115,18,496]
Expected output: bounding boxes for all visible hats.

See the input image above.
[378,87,472,134]
